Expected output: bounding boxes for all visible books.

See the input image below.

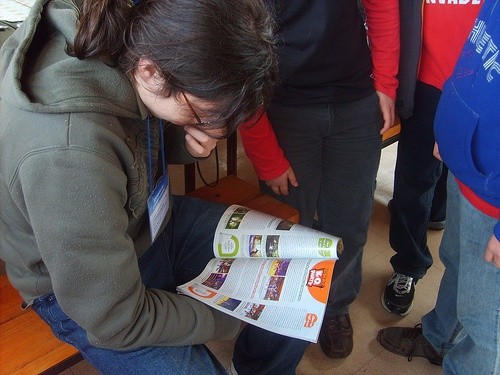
[176,202,345,344]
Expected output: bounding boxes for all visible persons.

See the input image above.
[236,0,499,374]
[0,0,315,375]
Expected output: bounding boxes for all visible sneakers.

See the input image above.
[381,272,419,317]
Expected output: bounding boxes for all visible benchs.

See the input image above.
[0,175,298,375]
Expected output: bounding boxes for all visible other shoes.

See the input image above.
[376,323,443,366]
[427,185,447,230]
[318,313,354,359]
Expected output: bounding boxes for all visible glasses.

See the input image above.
[182,93,228,126]
